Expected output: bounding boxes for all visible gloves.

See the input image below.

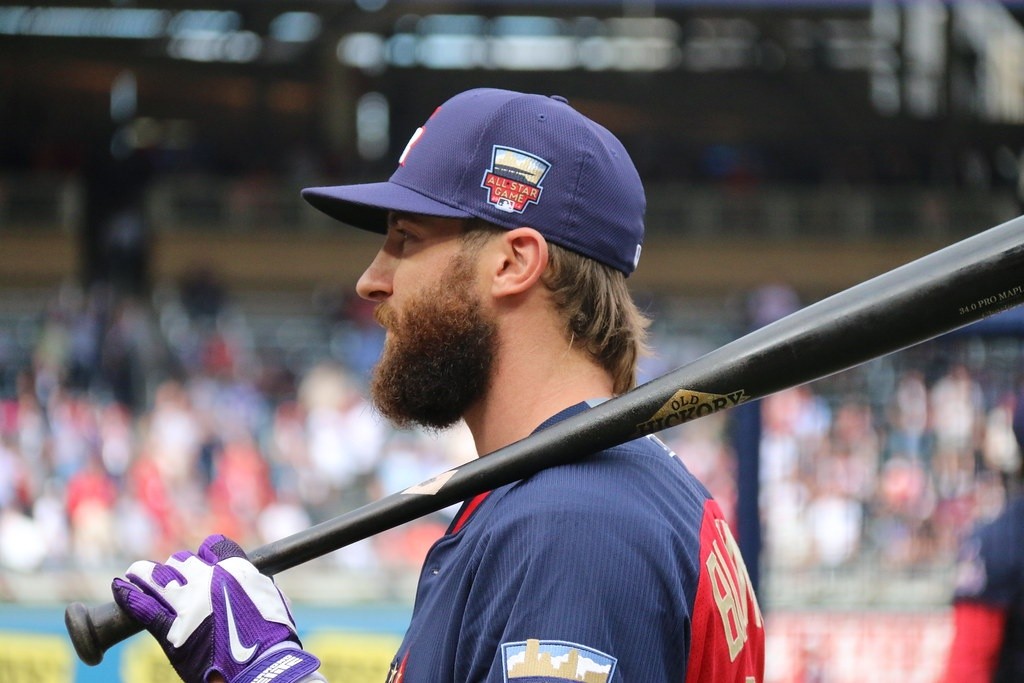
[111,535,321,683]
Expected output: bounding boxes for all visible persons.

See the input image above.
[113,85,767,683]
[940,410,1024,683]
[0,263,1024,610]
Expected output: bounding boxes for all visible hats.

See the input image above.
[301,89,647,278]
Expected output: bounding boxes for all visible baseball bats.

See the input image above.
[66,214,1024,667]
